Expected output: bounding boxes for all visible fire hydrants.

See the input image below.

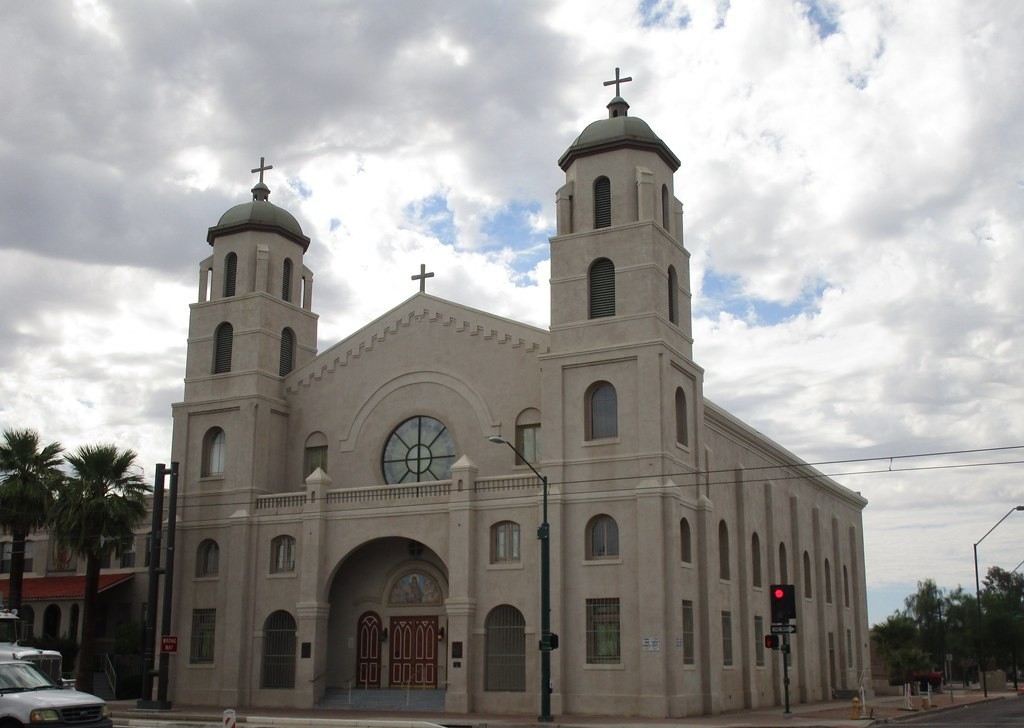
[851,696,865,720]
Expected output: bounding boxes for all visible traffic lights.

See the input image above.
[770,584,791,626]
[764,634,777,650]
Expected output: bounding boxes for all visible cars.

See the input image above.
[0,608,113,728]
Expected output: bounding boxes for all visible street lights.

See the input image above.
[489,435,557,721]
[973,506,1024,696]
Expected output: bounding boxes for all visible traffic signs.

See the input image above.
[771,625,797,634]
